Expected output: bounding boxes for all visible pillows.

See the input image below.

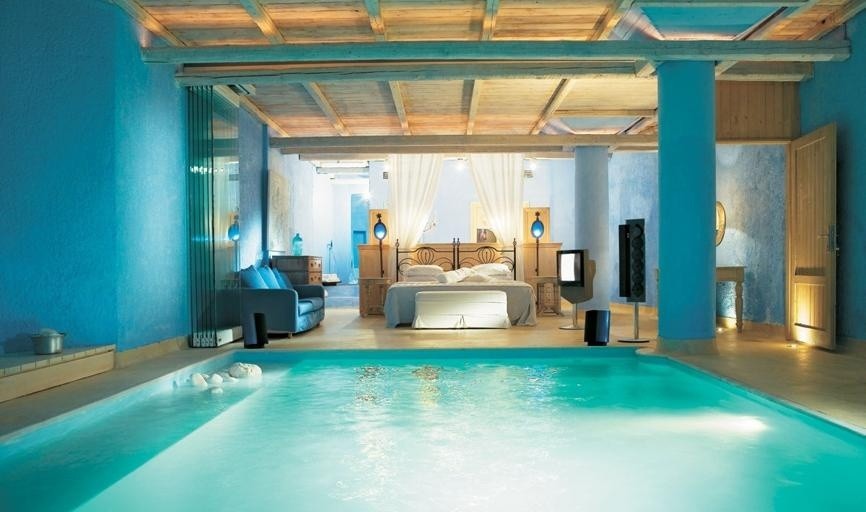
[237,266,288,290]
[402,263,511,283]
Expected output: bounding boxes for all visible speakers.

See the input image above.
[583,310,611,346]
[244,313,268,348]
[619,219,645,302]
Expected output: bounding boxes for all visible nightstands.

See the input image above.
[532,276,561,316]
[360,277,392,317]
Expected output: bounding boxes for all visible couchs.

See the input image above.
[241,272,325,339]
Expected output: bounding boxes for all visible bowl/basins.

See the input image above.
[30,331,65,354]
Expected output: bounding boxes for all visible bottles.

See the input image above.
[293,233,303,256]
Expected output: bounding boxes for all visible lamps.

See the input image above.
[373,212,387,278]
[530,212,545,276]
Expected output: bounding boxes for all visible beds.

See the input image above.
[385,237,535,327]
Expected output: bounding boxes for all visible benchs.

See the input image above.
[414,291,507,329]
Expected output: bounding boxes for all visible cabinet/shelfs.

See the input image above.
[273,255,323,285]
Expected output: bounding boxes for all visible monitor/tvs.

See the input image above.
[557,250,584,287]
